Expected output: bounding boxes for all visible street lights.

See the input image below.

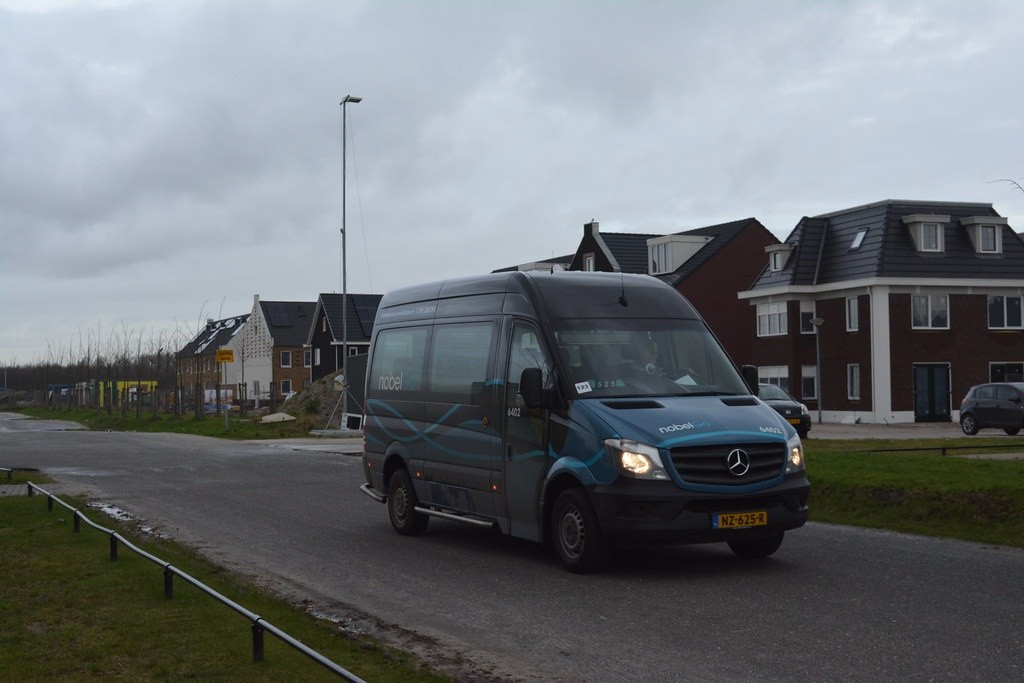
[810,317,824,423]
[338,94,362,425]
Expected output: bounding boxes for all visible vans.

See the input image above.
[361,270,813,572]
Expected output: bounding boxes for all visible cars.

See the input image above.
[757,382,812,439]
[959,381,1024,436]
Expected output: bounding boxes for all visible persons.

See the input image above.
[632,340,678,387]
